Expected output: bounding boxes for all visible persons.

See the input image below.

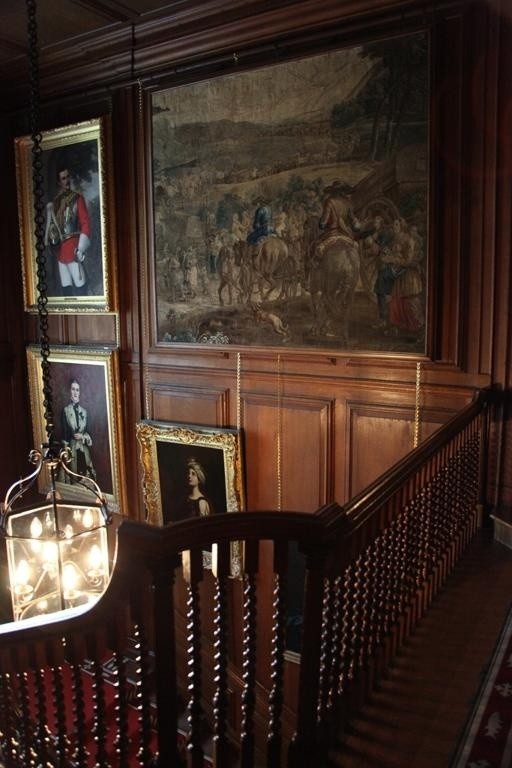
[166,456,214,522]
[57,380,98,490]
[44,165,91,296]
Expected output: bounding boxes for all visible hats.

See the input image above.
[321,179,358,196]
[186,455,209,485]
[250,195,271,207]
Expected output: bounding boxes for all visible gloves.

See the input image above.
[74,232,92,263]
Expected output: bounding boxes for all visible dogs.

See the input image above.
[249,301,292,344]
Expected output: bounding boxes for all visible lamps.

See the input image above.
[0,1,114,621]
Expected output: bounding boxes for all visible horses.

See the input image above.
[232,234,291,301]
[299,212,363,340]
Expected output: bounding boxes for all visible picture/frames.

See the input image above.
[27,346,127,516]
[135,421,244,581]
[143,17,440,364]
[11,114,121,317]
